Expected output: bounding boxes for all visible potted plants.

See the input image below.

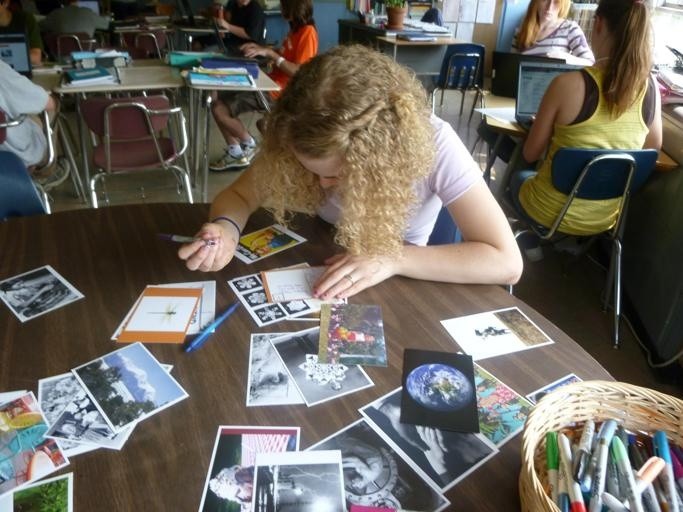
[384,0,408,31]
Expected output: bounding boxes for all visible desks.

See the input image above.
[374,36,466,106]
[0,200,618,512]
[470,93,679,202]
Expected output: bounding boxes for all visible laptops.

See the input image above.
[486,47,567,97]
[515,61,587,134]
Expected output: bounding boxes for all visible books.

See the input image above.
[363,1,451,43]
[181,56,260,87]
[32,49,131,87]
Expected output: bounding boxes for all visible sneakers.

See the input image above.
[209,137,260,170]
[526,245,542,262]
[40,158,70,190]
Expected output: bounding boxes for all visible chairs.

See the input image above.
[499,148,659,349]
[426,43,485,122]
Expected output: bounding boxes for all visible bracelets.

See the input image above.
[275,56,286,68]
[211,216,241,240]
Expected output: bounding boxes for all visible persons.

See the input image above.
[177,42,524,301]
[0,59,63,174]
[370,399,459,486]
[209,0,318,172]
[478,0,596,164]
[0,276,56,310]
[0,0,44,65]
[508,0,664,263]
[42,0,113,51]
[208,0,267,98]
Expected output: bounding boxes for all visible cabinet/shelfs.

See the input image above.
[337,16,451,58]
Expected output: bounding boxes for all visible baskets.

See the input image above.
[518,379,683,511]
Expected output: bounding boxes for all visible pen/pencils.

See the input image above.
[546,419,683,512]
[184,301,240,353]
[152,232,217,246]
